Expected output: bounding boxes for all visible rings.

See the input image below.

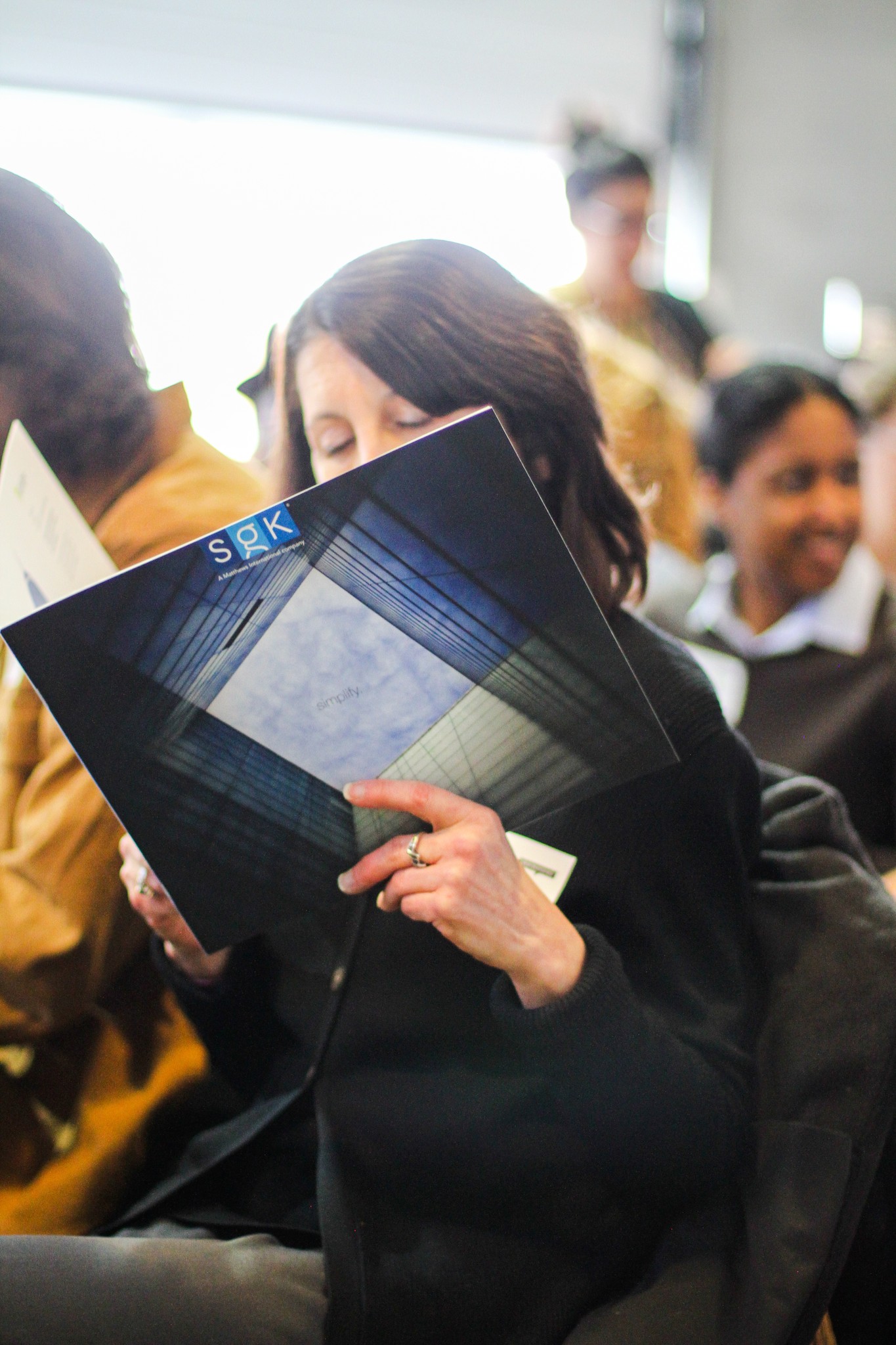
[408,828,426,868]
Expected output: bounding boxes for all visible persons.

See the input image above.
[550,127,741,620]
[0,238,764,1345]
[640,358,896,891]
[0,166,261,1245]
